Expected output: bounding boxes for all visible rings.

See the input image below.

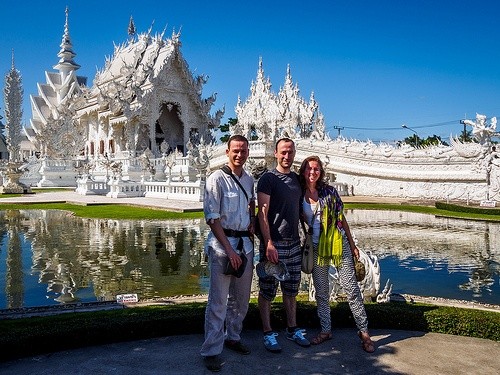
[230,257,233,260]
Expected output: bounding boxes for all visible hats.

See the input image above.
[223,253,248,278]
[256,258,290,282]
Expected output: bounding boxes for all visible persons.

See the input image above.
[299,156,377,354]
[200,136,255,372]
[256,138,311,349]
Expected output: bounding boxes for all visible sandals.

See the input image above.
[310,333,335,344]
[358,331,377,352]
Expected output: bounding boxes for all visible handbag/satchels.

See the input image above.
[301,233,313,274]
[352,254,365,281]
[253,212,278,242]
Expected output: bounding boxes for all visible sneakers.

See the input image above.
[204,355,221,371]
[261,331,281,350]
[285,328,310,347]
[224,339,251,355]
